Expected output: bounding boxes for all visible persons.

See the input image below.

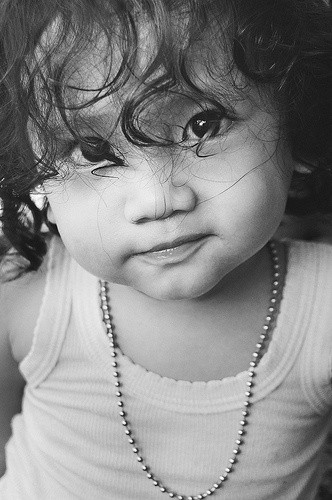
[0,0,332,499]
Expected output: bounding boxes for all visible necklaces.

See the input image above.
[96,239,280,499]
